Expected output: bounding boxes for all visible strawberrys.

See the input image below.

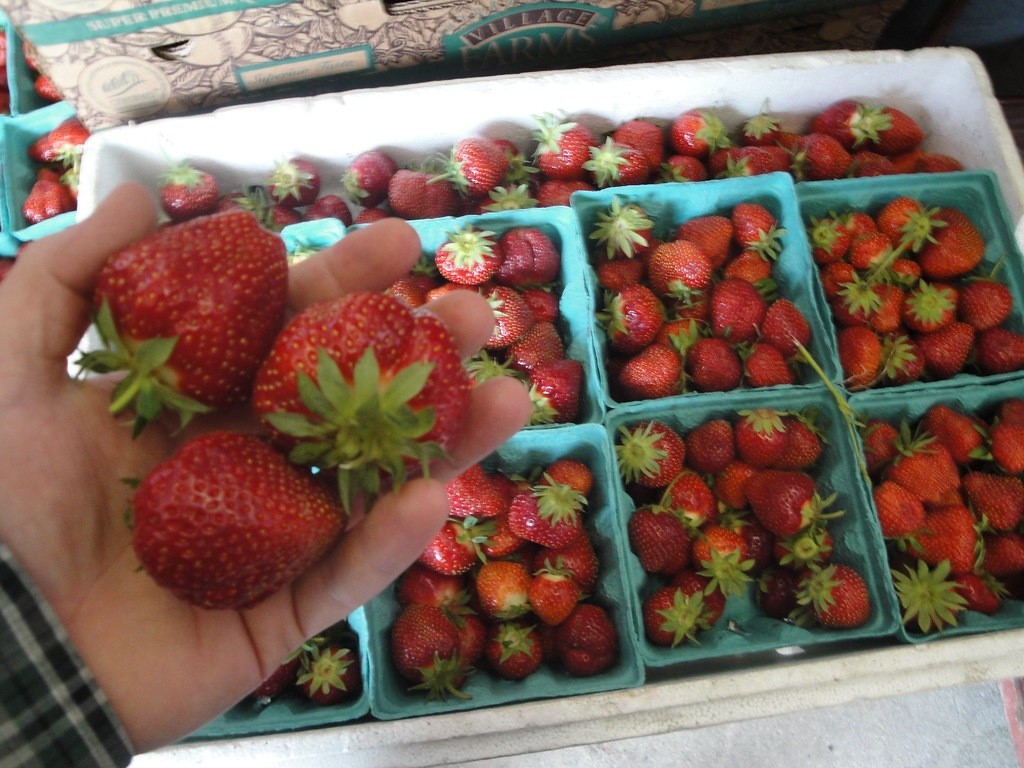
[0,26,1024,706]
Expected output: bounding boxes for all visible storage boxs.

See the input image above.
[0,0,1024,768]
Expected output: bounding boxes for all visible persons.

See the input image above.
[0,182,530,768]
